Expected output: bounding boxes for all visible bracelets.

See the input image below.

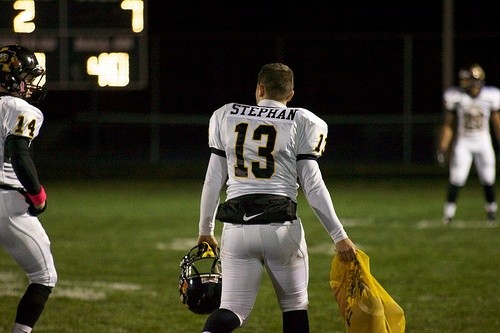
[27,185,46,207]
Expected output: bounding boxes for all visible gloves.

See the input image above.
[25,198,47,216]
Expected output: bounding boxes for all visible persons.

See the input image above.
[434,64,500,224]
[196,63,357,333]
[0,45,57,333]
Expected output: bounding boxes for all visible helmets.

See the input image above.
[458,63,485,87]
[0,45,44,101]
[178,243,222,315]
